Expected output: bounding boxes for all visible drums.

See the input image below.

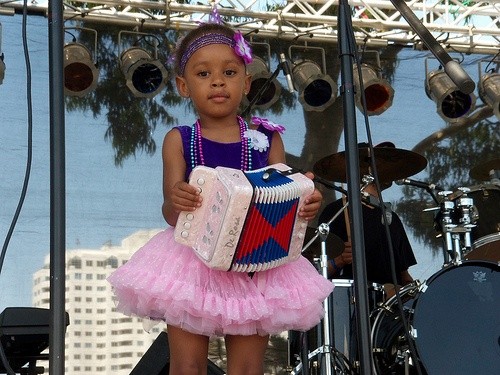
[288,277,389,375]
[436,183,500,263]
[370,259,500,375]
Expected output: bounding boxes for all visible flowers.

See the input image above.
[250,116,287,134]
[243,130,270,153]
[232,29,253,64]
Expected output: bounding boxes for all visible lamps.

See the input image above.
[289,40,338,113]
[63,20,99,100]
[352,47,395,117]
[118,25,168,98]
[424,56,478,123]
[476,58,500,121]
[245,37,281,109]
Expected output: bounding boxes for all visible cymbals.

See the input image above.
[315,144,428,185]
[302,226,345,260]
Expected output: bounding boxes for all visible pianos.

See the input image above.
[0,306,71,374]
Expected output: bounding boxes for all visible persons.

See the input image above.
[107,24,335,375]
[317,144,418,300]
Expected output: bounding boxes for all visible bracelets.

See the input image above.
[331,258,341,272]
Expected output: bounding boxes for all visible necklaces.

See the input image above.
[191,115,253,173]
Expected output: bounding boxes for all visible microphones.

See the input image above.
[279,49,294,93]
[395,178,444,191]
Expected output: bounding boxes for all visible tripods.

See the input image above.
[288,167,375,375]
